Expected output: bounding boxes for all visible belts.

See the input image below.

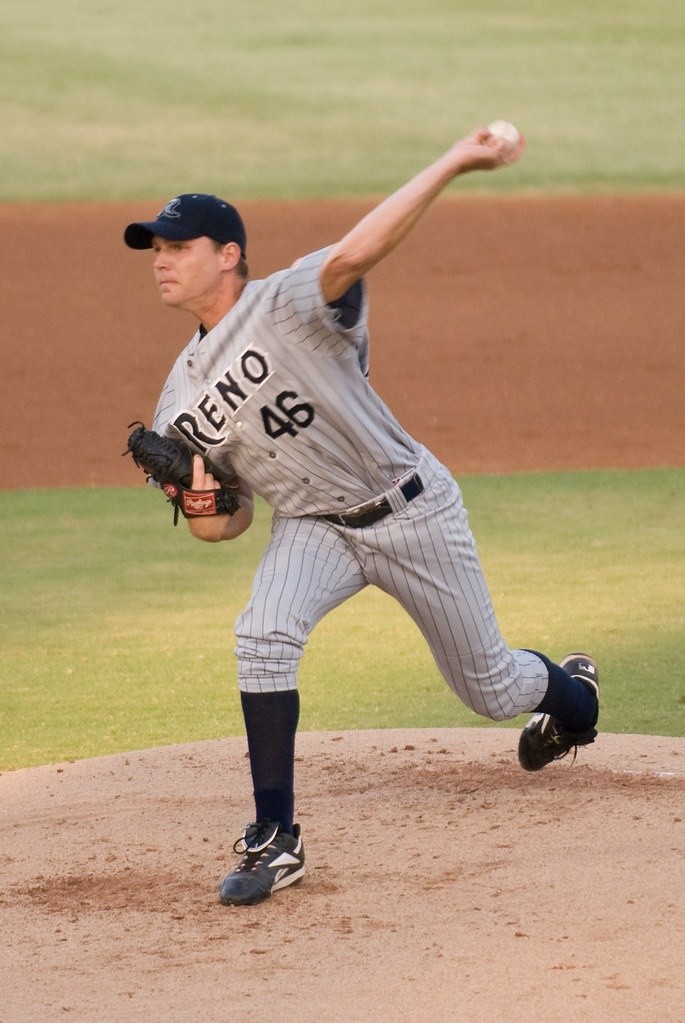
[321,472,425,530]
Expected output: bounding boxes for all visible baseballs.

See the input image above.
[487,121,520,152]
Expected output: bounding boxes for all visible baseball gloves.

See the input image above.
[127,427,242,519]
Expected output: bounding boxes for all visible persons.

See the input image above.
[122,128,600,905]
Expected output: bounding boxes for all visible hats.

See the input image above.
[123,193,245,258]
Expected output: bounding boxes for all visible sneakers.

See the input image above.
[219,822,307,905]
[518,652,599,770]
